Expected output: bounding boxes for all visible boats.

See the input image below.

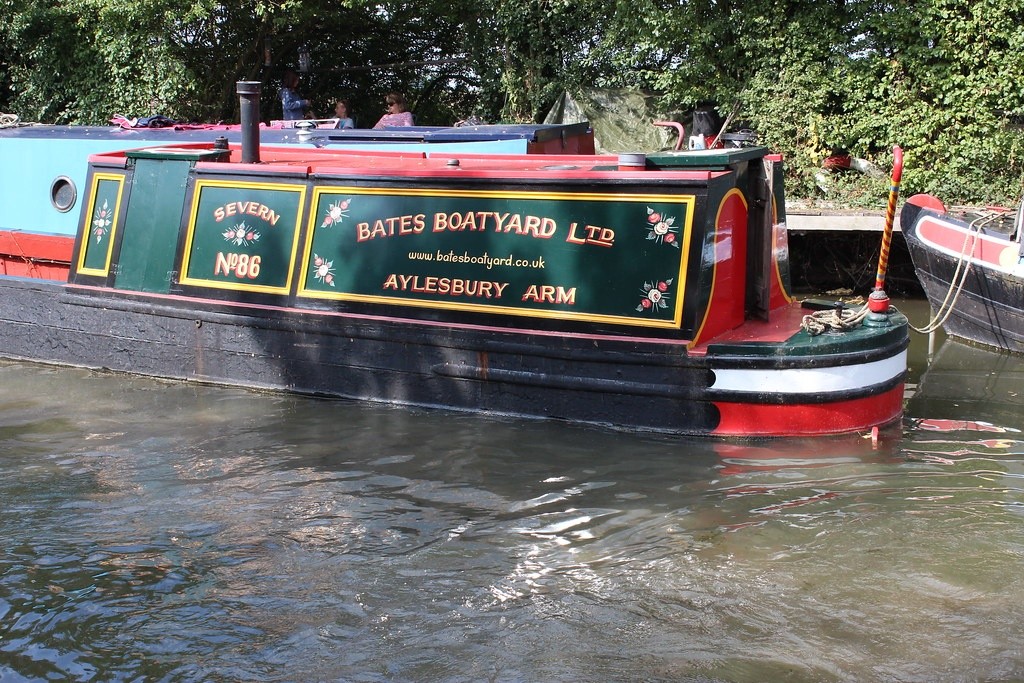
[900,175,1024,365]
[0,75,917,440]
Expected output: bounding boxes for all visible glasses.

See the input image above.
[385,100,397,107]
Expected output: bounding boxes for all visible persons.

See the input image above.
[372,92,413,127]
[281,69,311,119]
[332,99,354,129]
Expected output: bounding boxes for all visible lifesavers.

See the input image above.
[813,154,891,196]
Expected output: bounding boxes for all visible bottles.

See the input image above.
[688,133,705,150]
